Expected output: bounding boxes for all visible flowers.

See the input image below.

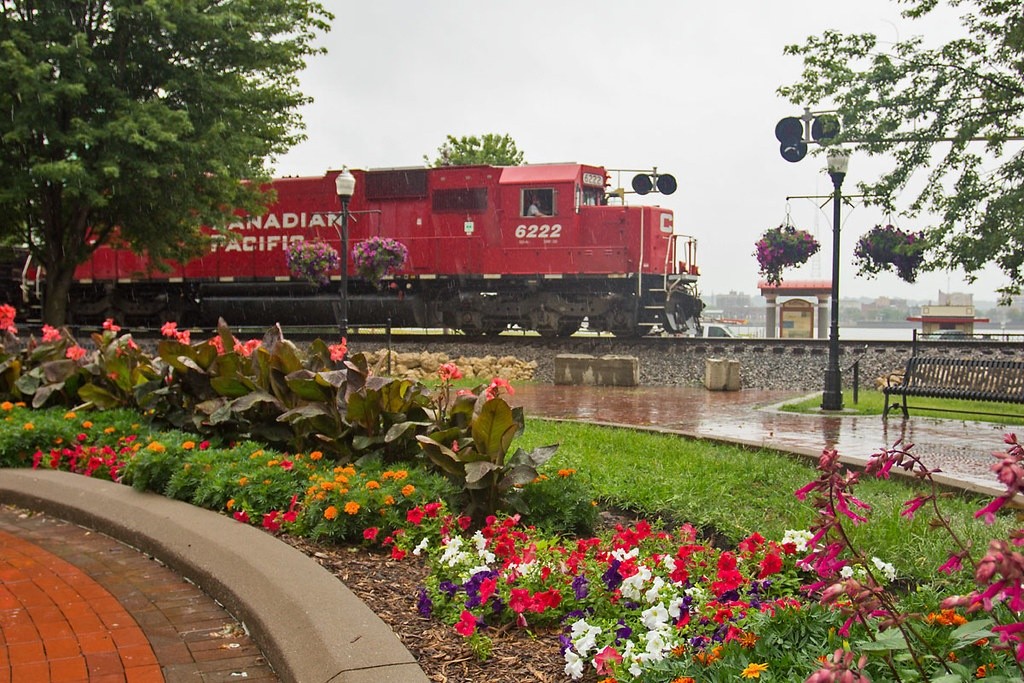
[351,235,409,290]
[750,224,821,287]
[851,224,930,284]
[285,240,341,290]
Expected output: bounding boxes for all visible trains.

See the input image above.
[8,160,709,338]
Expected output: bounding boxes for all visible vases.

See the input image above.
[872,248,894,262]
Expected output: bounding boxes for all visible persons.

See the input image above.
[527,194,547,217]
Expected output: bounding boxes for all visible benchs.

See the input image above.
[882,357,1024,420]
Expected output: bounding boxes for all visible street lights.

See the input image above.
[334,164,357,337]
[820,141,849,408]
